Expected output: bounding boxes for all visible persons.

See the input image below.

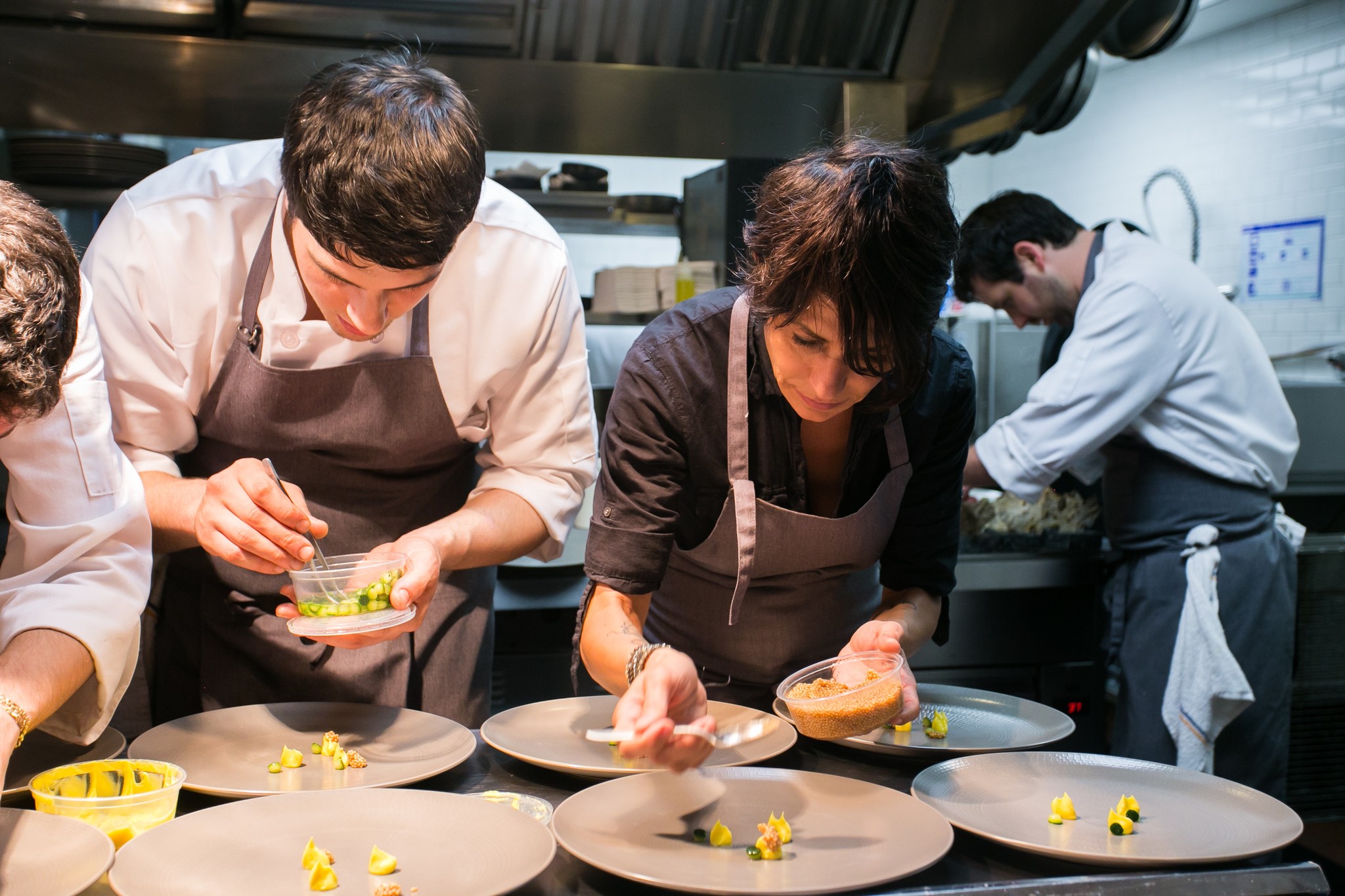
[68,53,603,735]
[572,137,984,775]
[948,188,1307,804]
[1,182,156,812]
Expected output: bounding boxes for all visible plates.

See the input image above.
[110,788,556,894]
[482,693,798,779]
[911,751,1305,865]
[125,701,477,795]
[1,720,125,803]
[775,682,1078,751]
[554,765,954,893]
[0,805,115,895]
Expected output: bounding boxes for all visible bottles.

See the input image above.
[675,252,696,303]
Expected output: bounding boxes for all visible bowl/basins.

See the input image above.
[29,757,187,883]
[775,648,905,742]
[287,550,405,619]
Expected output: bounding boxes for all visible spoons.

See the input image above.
[585,718,778,750]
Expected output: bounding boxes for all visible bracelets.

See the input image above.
[1,695,30,748]
[626,641,673,691]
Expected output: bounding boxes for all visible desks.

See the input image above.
[395,724,1336,896]
